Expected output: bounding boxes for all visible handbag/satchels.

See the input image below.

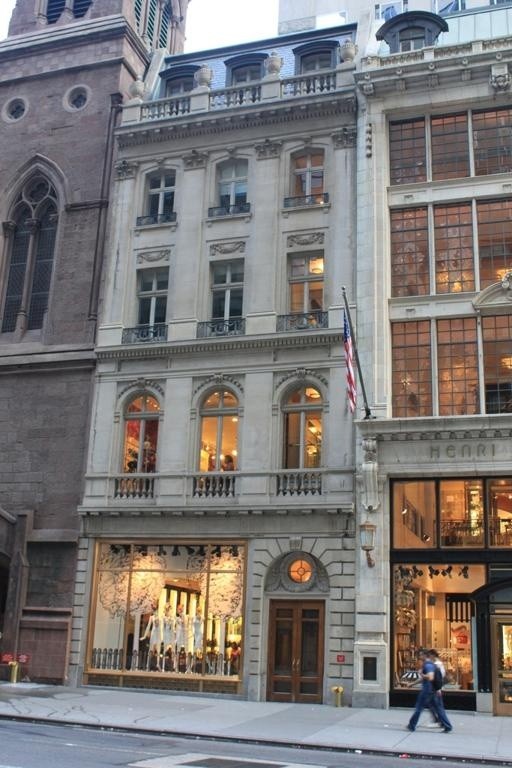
[434,668,442,691]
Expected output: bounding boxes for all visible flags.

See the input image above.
[344,308,356,415]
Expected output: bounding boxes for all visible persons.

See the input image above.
[230,641,241,675]
[402,649,453,734]
[140,604,219,674]
[428,649,446,729]
[222,455,235,471]
